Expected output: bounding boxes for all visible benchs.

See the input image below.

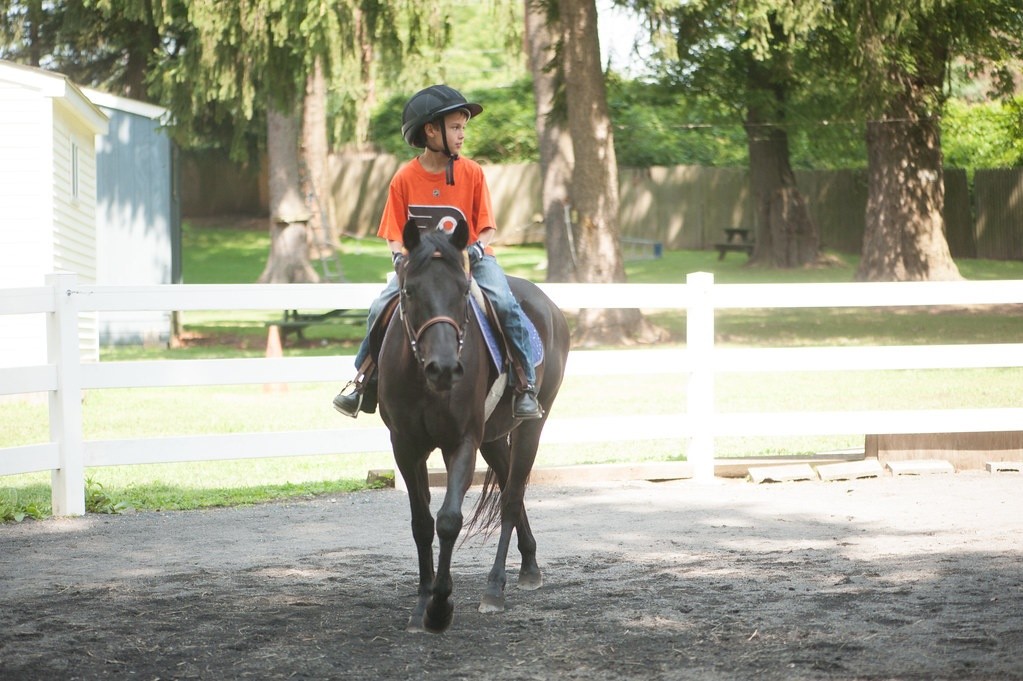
[714,242,755,260]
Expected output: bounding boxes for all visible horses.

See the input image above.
[377,218,571,635]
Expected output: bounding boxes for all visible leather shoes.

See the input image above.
[333,387,361,418]
[515,389,539,417]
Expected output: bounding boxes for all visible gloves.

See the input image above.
[467,246,481,269]
[392,252,407,275]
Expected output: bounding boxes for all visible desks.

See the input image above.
[717,228,753,259]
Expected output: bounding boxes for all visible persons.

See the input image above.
[333,85,545,419]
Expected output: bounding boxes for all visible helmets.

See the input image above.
[401,85,483,149]
